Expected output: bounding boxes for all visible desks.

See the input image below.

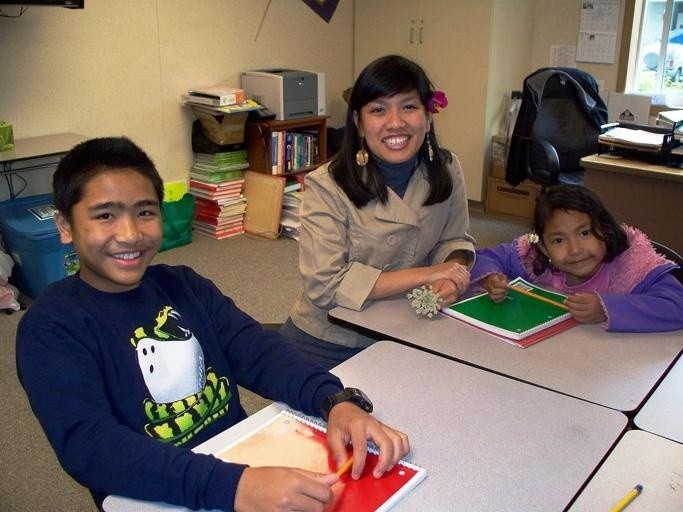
[1,132,99,202]
[579,154,683,275]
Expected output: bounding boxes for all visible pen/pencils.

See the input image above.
[612,485,642,512]
[335,456,353,477]
[509,286,569,309]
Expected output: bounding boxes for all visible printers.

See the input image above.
[241,68,326,120]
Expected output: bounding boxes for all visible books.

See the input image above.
[182,98,321,242]
[598,126,671,153]
[441,276,573,341]
[211,408,430,512]
[438,311,578,349]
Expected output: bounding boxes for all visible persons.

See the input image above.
[279,54,478,371]
[15,136,410,511]
[468,182,683,333]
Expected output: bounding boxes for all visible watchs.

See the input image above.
[319,387,373,421]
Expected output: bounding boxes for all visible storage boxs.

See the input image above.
[490,135,509,179]
[485,178,542,229]
[0,193,78,301]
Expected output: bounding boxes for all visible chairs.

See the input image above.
[518,68,607,188]
[650,241,683,270]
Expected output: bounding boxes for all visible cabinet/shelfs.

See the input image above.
[248,115,330,187]
[353,0,537,204]
